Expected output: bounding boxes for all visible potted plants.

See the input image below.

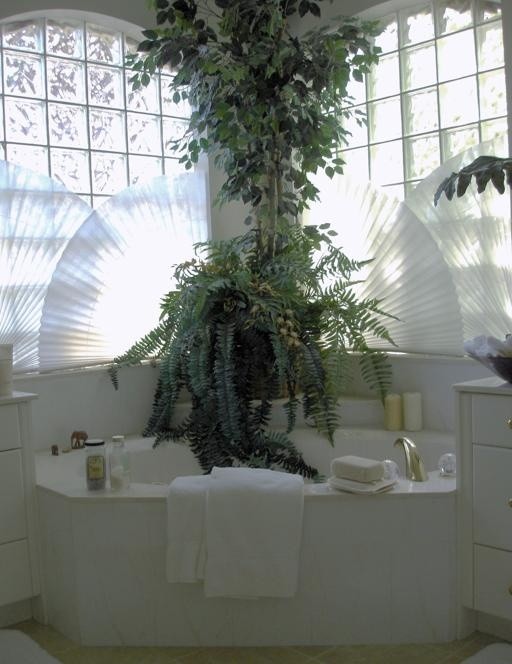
[106,0,405,483]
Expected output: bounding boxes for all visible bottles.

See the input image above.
[83,440,108,494]
[0,343,13,397]
[109,437,132,491]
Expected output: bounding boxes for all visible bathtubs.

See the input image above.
[35,423,475,644]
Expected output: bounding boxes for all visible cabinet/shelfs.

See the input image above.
[0,390,49,630]
[452,375,512,644]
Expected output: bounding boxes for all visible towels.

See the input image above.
[195,466,304,599]
[166,474,209,584]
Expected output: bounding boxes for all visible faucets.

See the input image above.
[394,436,428,482]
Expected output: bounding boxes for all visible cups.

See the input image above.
[383,391,422,431]
